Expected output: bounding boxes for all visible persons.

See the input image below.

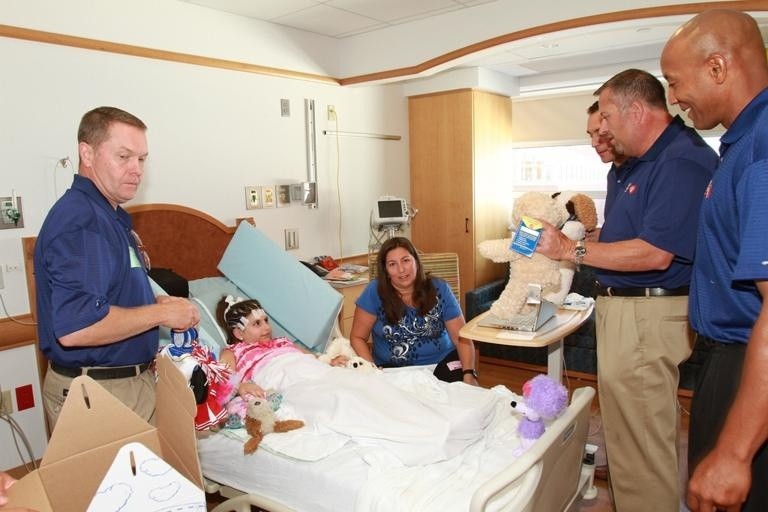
[586,101,635,217]
[350,237,479,386]
[515,69,719,512]
[660,11,768,512]
[216,294,351,402]
[34,106,200,433]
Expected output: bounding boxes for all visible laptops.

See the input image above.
[475,296,552,333]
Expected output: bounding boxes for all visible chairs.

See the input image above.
[370,252,460,307]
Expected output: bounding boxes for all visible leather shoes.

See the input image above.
[596,464,608,480]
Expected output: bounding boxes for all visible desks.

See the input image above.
[459,291,594,398]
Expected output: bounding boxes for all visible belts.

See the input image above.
[50,362,151,380]
[593,281,691,297]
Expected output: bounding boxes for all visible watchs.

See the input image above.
[573,240,586,269]
[463,369,478,380]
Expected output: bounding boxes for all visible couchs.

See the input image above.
[457,276,596,378]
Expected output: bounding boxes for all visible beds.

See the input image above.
[19,202,597,512]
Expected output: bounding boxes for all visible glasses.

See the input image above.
[130,229,151,271]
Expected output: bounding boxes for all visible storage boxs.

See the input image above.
[0,353,206,512]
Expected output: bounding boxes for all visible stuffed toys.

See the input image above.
[477,192,598,318]
[208,394,305,456]
[510,373,570,441]
[318,337,377,370]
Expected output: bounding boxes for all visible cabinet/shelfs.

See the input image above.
[329,282,368,339]
[409,89,475,312]
